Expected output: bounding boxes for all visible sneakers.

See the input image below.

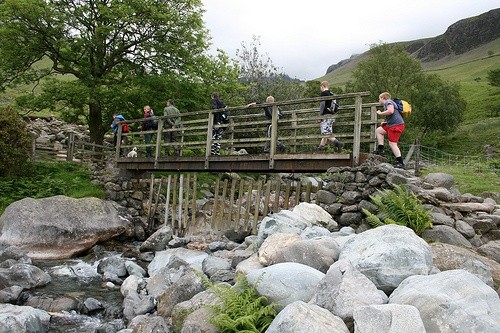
[313,146,326,154]
[393,161,405,169]
[278,143,287,154]
[334,140,344,154]
[373,147,385,155]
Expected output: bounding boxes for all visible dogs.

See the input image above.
[127,147,138,158]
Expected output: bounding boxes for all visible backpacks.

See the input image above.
[151,114,158,130]
[393,98,412,119]
[121,123,129,133]
[218,111,229,125]
[329,94,339,114]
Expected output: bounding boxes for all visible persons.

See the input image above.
[209,92,226,156]
[163,99,181,157]
[317,81,343,152]
[137,105,155,157]
[373,92,404,168]
[110,113,128,156]
[247,96,285,154]
